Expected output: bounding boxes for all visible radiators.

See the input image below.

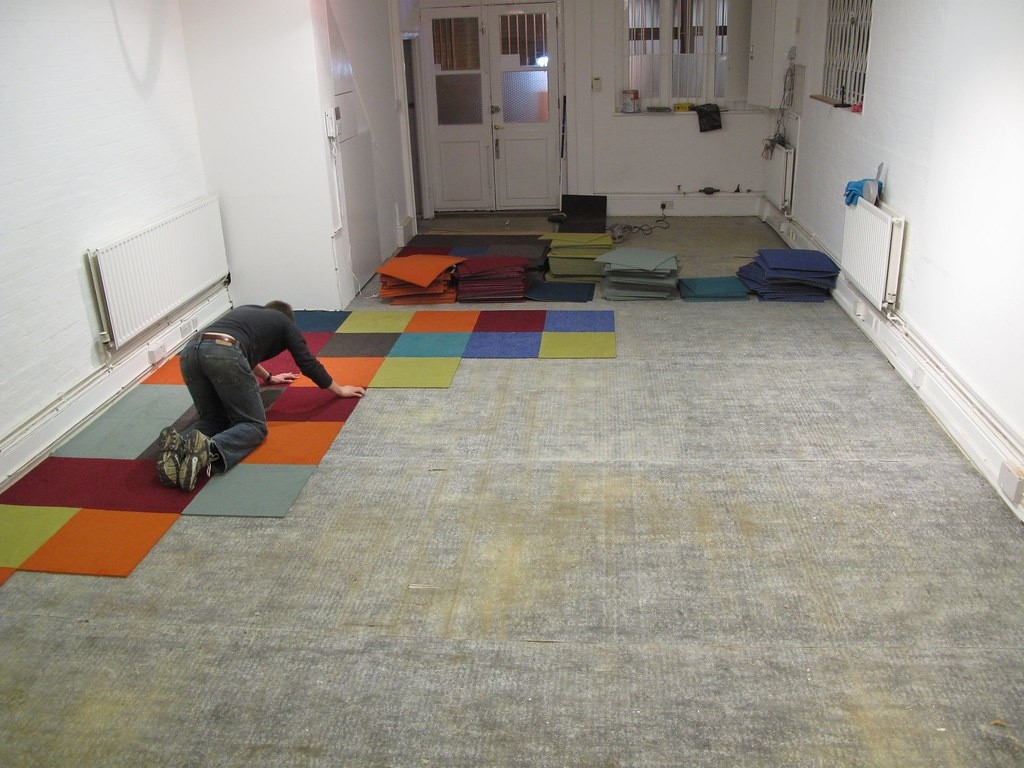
[761,138,796,213]
[84,193,228,352]
[839,191,906,313]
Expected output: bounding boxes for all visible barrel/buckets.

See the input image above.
[621,89,639,113]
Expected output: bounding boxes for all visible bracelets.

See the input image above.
[265,372,273,384]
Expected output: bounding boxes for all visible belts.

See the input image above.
[191,333,236,345]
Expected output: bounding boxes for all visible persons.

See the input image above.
[155,302,368,493]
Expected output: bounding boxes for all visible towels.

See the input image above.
[842,179,883,208]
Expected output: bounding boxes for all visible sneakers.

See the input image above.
[157,425,215,492]
